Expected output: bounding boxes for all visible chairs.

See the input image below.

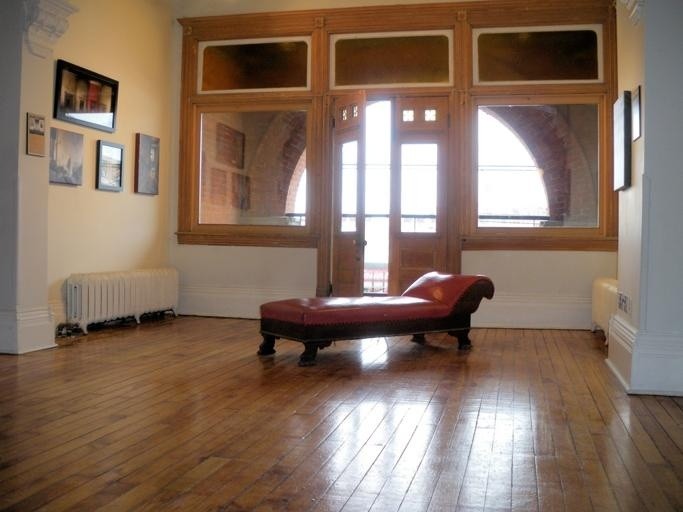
[256,271,494,368]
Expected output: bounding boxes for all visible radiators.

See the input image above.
[590,276,618,345]
[66,267,181,335]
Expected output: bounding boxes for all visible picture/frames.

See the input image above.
[51,58,119,134]
[94,140,125,194]
